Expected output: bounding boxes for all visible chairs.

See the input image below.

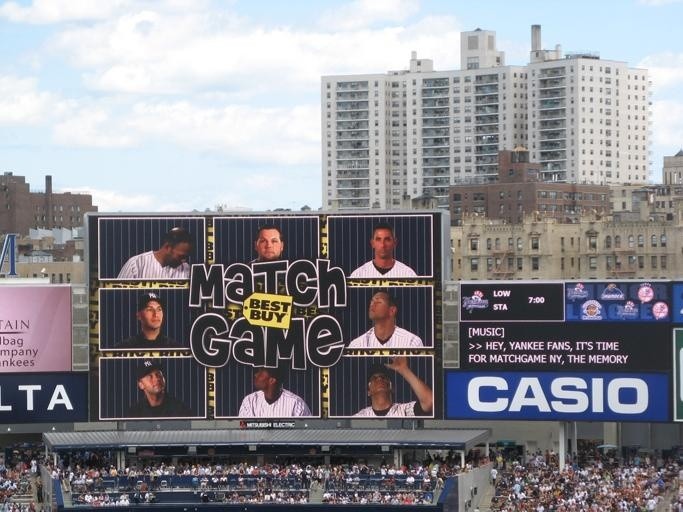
[11,473,450,512]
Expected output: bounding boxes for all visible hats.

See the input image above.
[368,364,390,379]
[136,359,164,379]
[135,290,163,312]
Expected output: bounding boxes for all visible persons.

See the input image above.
[0,437,682,512]
[236,358,314,417]
[122,358,193,416]
[245,222,292,269]
[114,226,195,280]
[350,355,432,416]
[112,292,188,348]
[347,290,425,348]
[345,222,418,277]
